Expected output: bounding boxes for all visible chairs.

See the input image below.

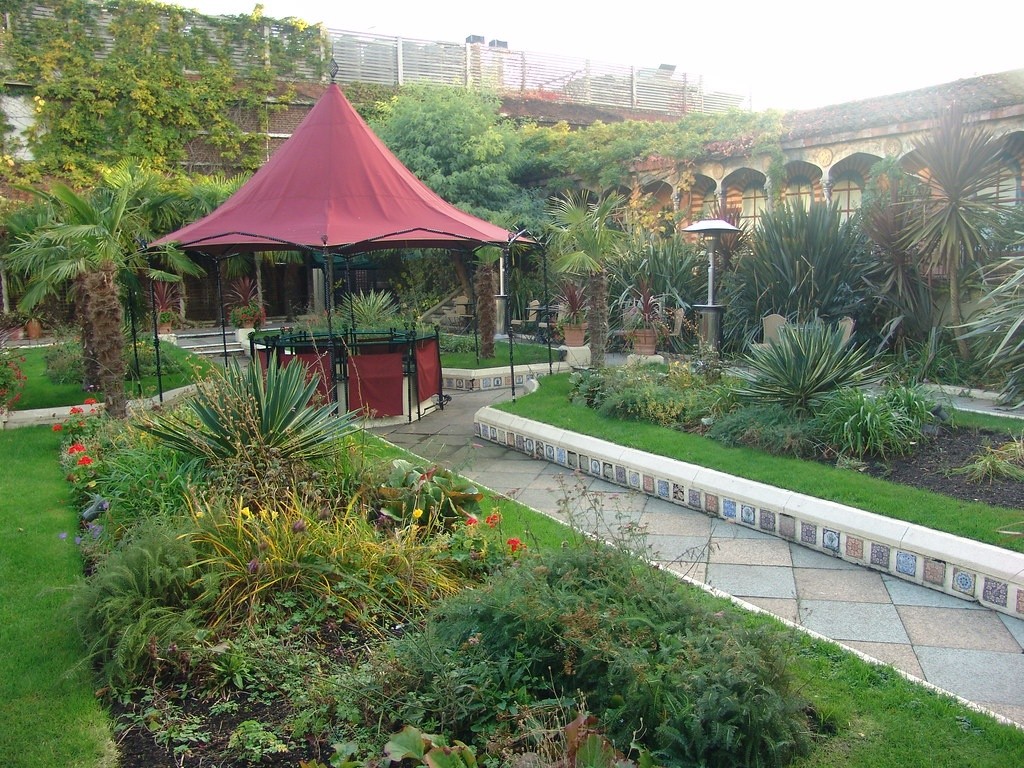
[511,299,558,340]
[837,316,857,344]
[761,314,788,348]
[447,296,470,335]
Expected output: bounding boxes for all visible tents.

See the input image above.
[145,60,553,407]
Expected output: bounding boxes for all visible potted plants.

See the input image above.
[630,276,660,352]
[555,279,593,344]
[0,309,26,341]
[27,310,47,338]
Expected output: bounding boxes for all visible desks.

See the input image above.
[456,302,478,334]
[524,307,553,335]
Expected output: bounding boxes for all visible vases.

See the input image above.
[157,321,172,333]
[238,318,262,328]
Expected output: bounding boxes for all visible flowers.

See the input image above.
[235,307,268,325]
[156,310,182,326]
